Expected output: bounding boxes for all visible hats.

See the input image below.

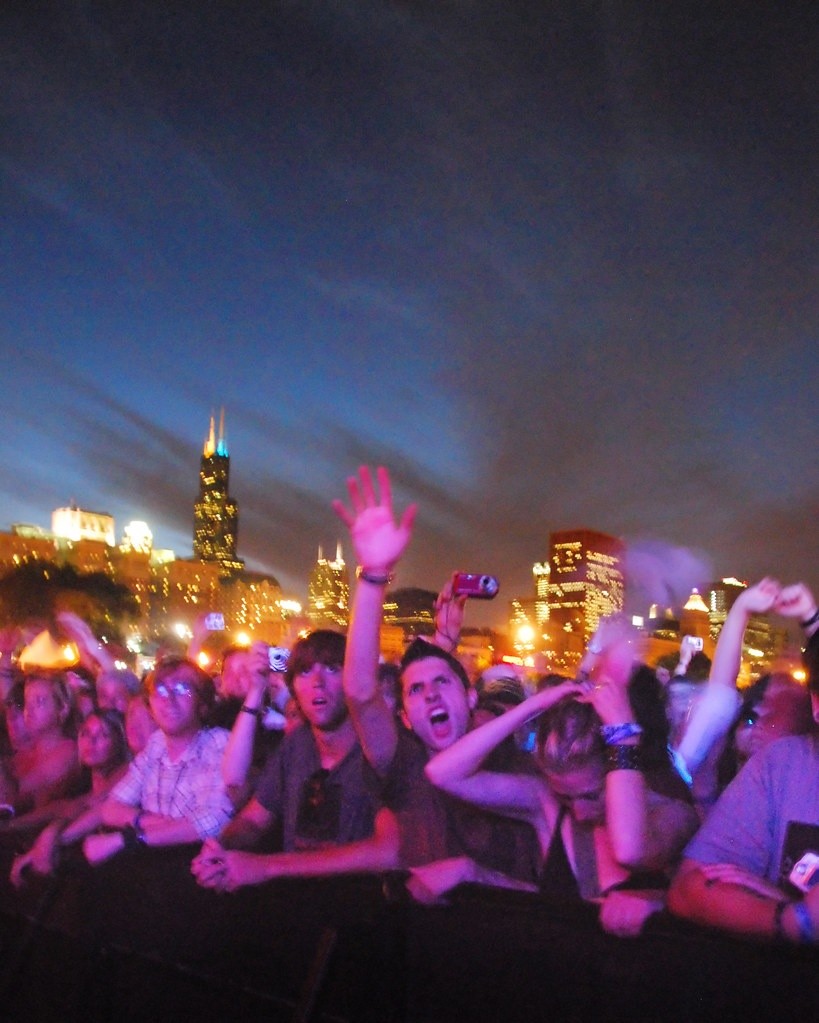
[475,664,525,703]
[747,672,807,715]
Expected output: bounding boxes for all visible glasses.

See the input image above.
[294,768,330,838]
[739,708,795,735]
[148,679,199,699]
[355,869,411,927]
[1,697,24,711]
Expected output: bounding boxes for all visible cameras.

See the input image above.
[452,573,500,599]
[20,864,56,887]
[629,615,655,632]
[266,646,291,672]
[790,853,819,892]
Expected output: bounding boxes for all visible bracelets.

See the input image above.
[602,745,641,774]
[359,572,389,585]
[799,610,819,629]
[795,902,814,943]
[134,810,146,831]
[240,705,258,716]
[773,900,789,934]
[599,722,644,745]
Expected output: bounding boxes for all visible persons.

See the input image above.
[0,466,818,945]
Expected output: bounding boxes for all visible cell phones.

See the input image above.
[688,637,703,652]
